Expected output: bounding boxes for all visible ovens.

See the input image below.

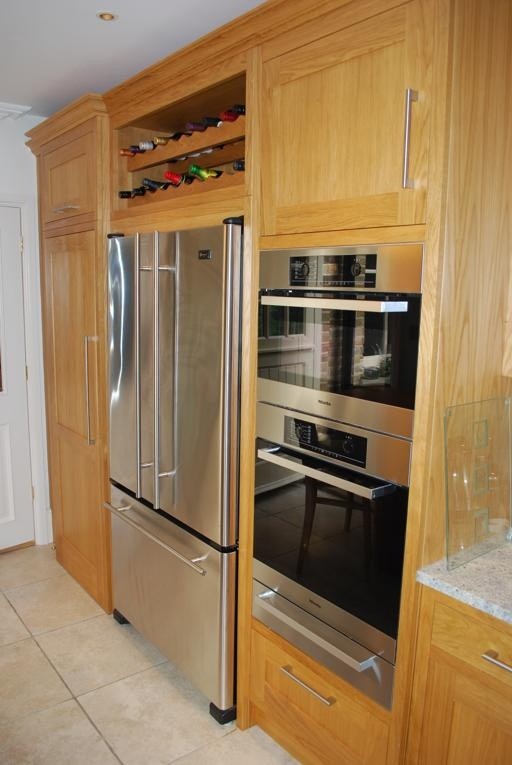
[251,403,409,708]
[256,246,421,439]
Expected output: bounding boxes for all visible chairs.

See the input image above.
[295,419,374,579]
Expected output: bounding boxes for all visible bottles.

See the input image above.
[119,106,245,199]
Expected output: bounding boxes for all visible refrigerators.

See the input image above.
[105,217,243,714]
